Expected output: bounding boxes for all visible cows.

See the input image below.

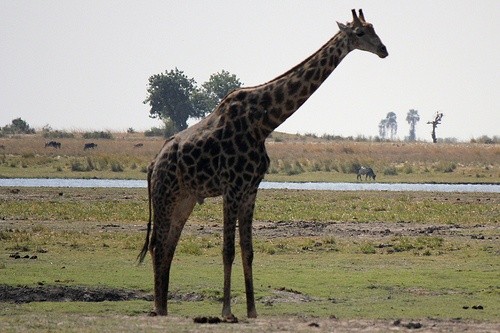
[355,167,376,181]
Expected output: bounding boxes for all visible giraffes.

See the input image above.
[132,9,388,323]
